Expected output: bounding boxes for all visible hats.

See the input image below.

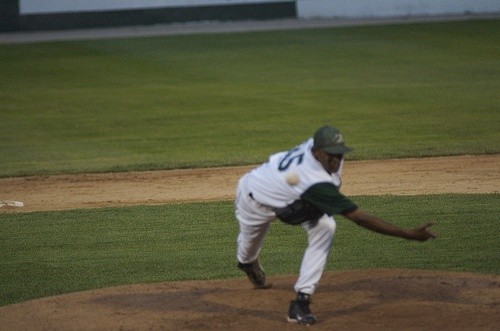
[313,124,355,154]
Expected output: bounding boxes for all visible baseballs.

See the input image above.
[286,171,301,186]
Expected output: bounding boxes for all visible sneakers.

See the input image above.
[238,255,266,288]
[286,293,316,325]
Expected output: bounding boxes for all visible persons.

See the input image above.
[233,126,436,327]
